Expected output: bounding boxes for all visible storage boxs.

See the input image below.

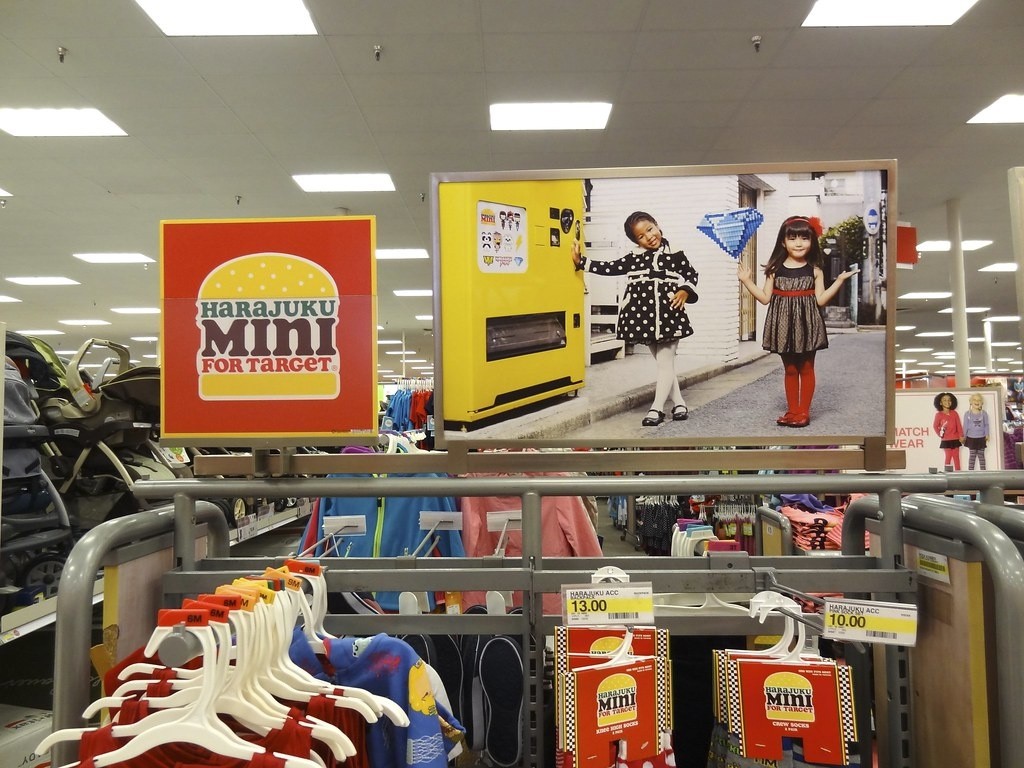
[0,704,53,768]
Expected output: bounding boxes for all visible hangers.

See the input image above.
[569,567,834,675]
[378,430,429,453]
[396,379,434,395]
[34,561,412,767]
[644,492,758,557]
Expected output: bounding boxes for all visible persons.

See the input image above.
[573,212,699,426]
[962,394,990,470]
[739,217,861,427]
[934,392,964,470]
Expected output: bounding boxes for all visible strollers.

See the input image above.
[1,329,320,612]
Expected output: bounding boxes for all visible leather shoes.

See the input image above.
[642,409,666,426]
[671,405,689,421]
[777,412,810,427]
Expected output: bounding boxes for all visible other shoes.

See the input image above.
[399,604,537,767]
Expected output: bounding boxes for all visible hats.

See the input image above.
[934,393,958,411]
